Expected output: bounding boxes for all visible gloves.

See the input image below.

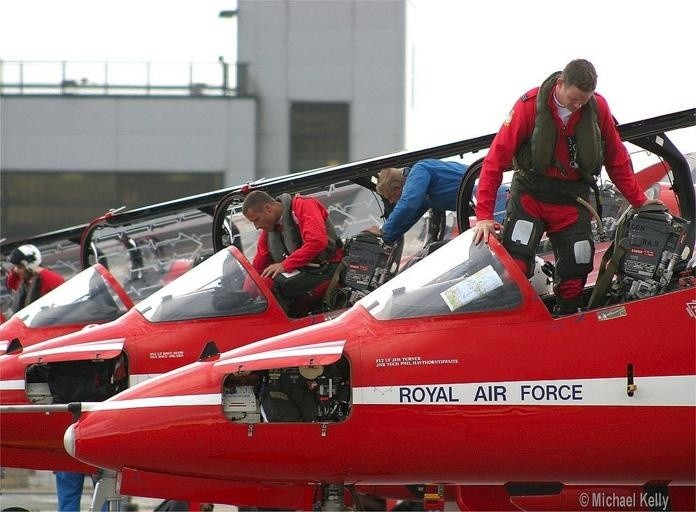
[21,259,36,273]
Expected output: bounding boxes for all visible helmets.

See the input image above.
[11,244,42,272]
[528,256,551,296]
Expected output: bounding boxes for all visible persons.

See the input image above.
[472,59,663,318]
[241,190,344,318]
[5,244,65,313]
[361,158,510,244]
[55,470,119,512]
